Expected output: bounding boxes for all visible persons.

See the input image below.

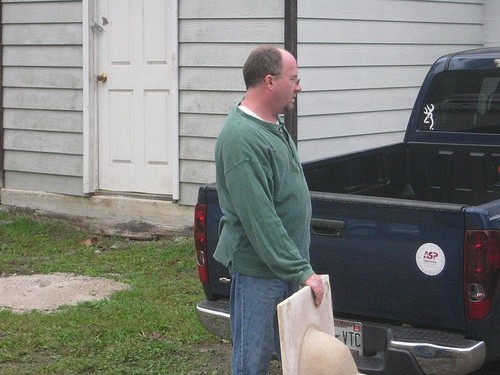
[212,44,324,375]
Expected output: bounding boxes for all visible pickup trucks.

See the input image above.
[193,47,500,374]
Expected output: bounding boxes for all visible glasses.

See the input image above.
[274,73,302,86]
